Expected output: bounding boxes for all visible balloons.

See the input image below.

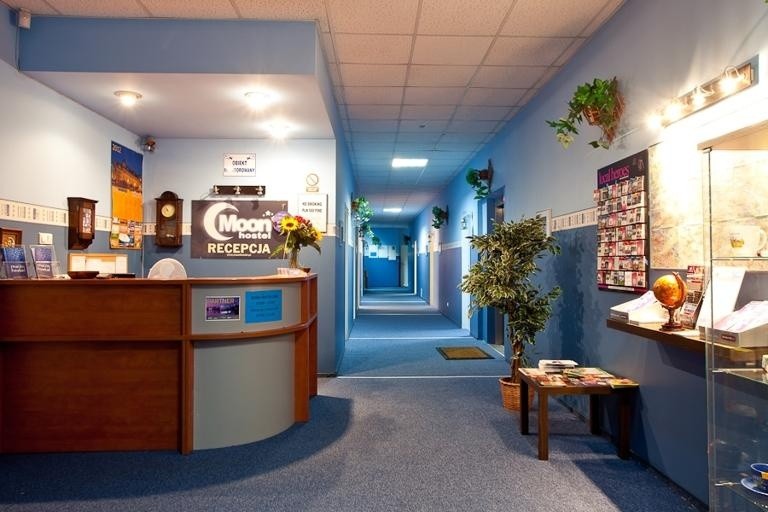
[154,190,184,247]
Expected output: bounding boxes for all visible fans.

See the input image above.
[191,199,287,259]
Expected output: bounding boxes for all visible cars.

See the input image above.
[750,462,768,487]
[730,224,767,258]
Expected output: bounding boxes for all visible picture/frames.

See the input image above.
[515,367,640,460]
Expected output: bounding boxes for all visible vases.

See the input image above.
[267,210,322,260]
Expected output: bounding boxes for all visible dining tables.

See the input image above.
[351,196,374,223]
[454,213,564,413]
[544,77,625,150]
[431,205,449,229]
[466,157,494,199]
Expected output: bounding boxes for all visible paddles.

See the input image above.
[435,346,496,360]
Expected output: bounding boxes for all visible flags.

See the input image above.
[289,244,301,268]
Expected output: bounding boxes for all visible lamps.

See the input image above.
[147,258,188,279]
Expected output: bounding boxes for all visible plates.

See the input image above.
[696,119,768,511]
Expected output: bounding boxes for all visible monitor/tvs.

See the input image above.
[596,175,645,288]
[680,265,705,323]
[521,360,640,389]
[2,247,28,279]
[31,246,53,278]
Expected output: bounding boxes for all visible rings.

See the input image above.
[568,376,585,378]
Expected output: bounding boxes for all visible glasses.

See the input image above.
[646,63,754,133]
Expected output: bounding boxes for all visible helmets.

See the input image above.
[68,271,100,279]
[740,477,768,497]
